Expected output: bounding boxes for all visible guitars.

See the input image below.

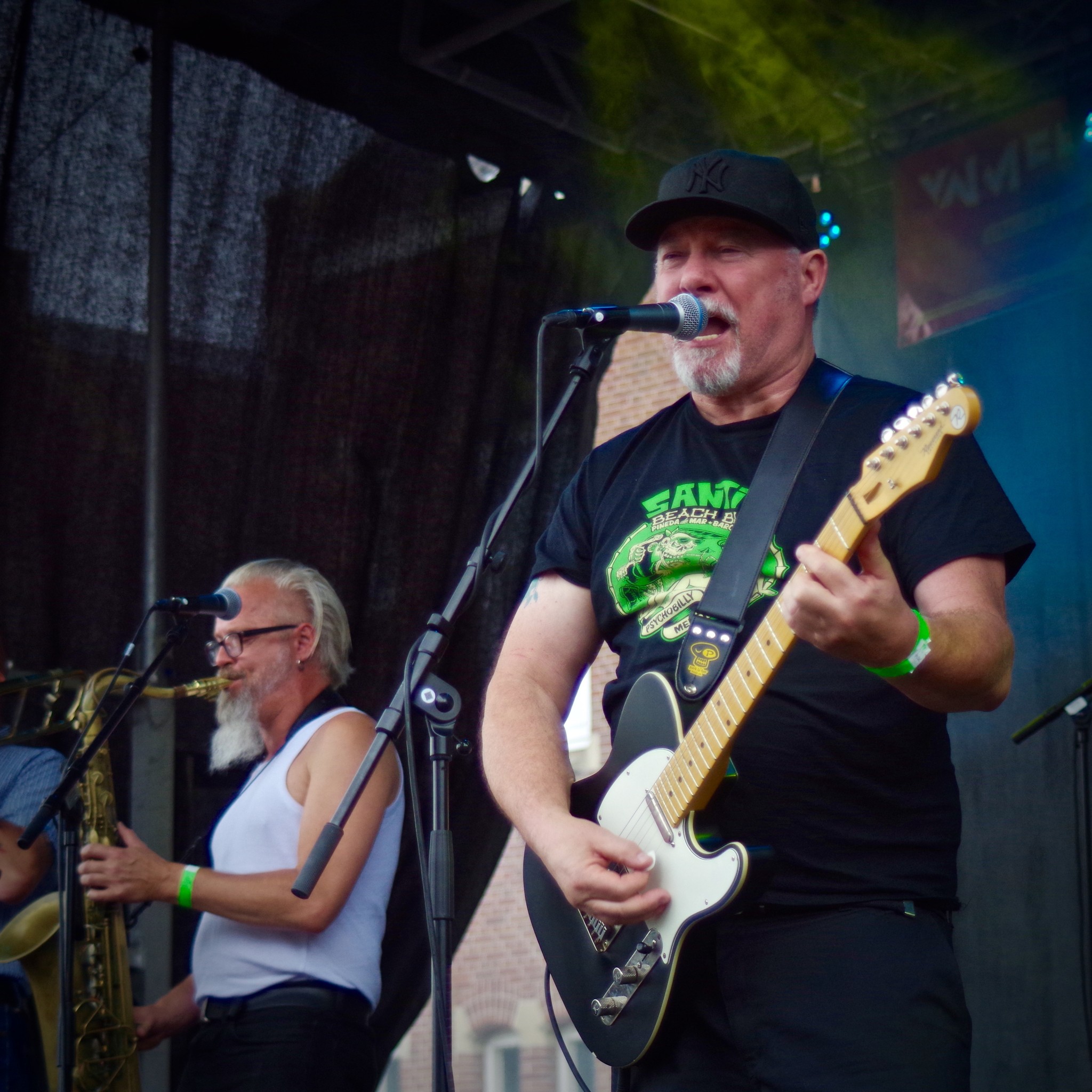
[522,368,984,1069]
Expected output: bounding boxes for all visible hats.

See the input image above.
[626,150,821,255]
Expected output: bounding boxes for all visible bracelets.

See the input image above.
[177,864,201,908]
[859,609,931,678]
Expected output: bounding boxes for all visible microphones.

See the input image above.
[154,588,242,621]
[544,292,708,342]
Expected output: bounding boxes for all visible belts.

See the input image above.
[197,987,329,1026]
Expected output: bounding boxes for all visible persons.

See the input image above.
[0,717,67,1092]
[76,558,406,1091]
[479,151,1035,1092]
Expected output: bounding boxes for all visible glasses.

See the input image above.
[205,623,299,666]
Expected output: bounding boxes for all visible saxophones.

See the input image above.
[0,666,237,1092]
